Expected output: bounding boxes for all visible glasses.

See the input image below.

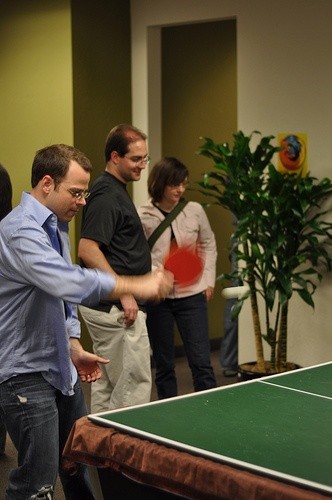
[52,177,91,200]
[120,154,151,166]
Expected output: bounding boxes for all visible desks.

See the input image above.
[61,361,332,500]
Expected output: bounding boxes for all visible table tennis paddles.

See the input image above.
[162,251,204,287]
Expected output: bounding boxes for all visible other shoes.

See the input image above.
[224,369,237,377]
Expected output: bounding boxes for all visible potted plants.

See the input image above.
[187,130,332,382]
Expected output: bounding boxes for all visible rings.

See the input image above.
[86,374,91,379]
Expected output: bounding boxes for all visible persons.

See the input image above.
[137,158,218,401]
[0,143,175,500]
[220,216,239,377]
[77,124,152,413]
[0,163,12,465]
